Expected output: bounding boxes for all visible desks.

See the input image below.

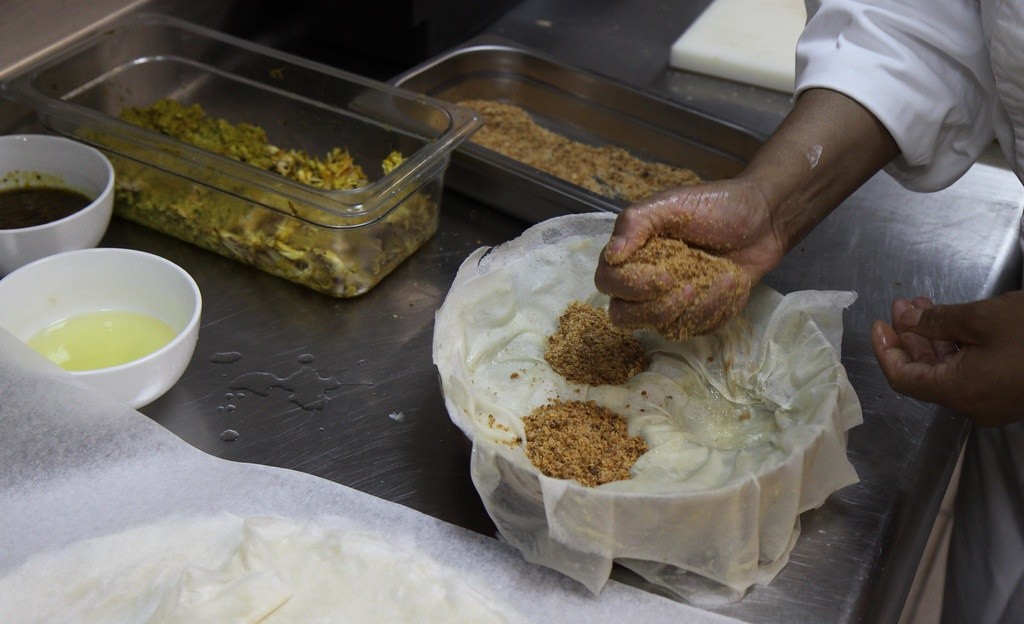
[0,0,1024,623]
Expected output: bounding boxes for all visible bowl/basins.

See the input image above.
[478,242,839,509]
[0,247,202,411]
[0,133,115,281]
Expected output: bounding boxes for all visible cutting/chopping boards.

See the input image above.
[669,0,806,95]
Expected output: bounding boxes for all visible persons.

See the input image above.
[594,0,1024,624]
